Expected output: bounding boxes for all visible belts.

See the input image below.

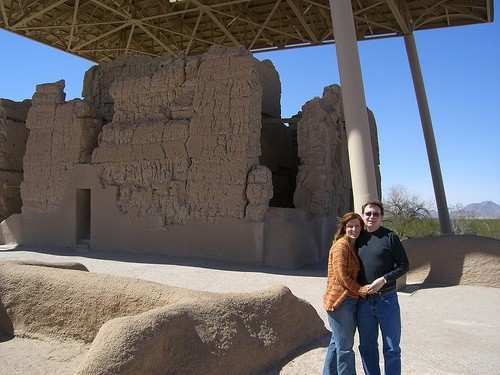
[364,287,397,297]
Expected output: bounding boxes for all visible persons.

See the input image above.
[347,199,410,375]
[319,212,376,375]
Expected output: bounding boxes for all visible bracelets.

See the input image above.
[381,276,387,283]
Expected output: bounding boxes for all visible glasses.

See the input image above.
[363,212,382,218]
